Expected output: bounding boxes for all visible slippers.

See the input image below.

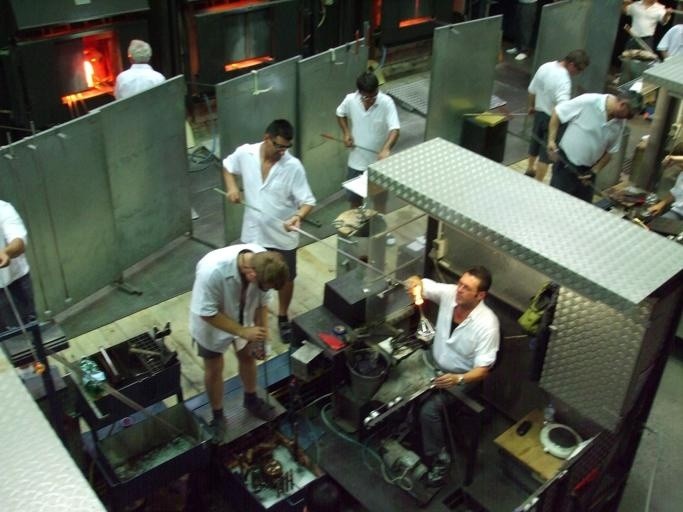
[279,322,291,343]
[252,341,266,360]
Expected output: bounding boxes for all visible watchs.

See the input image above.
[294,214,305,219]
[457,375,465,388]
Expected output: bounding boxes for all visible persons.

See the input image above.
[406,264,500,486]
[222,119,316,363]
[645,141,683,224]
[0,199,38,336]
[114,39,167,101]
[618,0,674,83]
[657,21,683,60]
[505,0,538,61]
[524,48,588,180]
[547,90,647,204]
[336,68,401,214]
[191,242,291,440]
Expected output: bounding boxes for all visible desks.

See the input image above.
[494,407,567,482]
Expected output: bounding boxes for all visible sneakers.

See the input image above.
[426,461,452,487]
[208,417,227,445]
[243,398,278,421]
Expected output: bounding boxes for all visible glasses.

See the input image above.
[456,276,481,298]
[577,66,584,71]
[272,140,293,148]
[361,90,378,100]
[625,111,634,119]
[257,282,269,292]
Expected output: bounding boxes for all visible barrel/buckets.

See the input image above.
[346,348,391,401]
